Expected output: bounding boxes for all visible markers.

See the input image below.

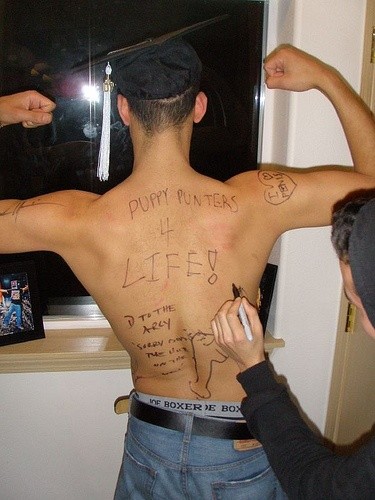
[231,280,255,342]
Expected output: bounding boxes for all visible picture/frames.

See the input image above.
[0,265,45,346]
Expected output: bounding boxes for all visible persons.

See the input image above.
[0,40,375,500]
[0,281,32,329]
[211,187,375,500]
